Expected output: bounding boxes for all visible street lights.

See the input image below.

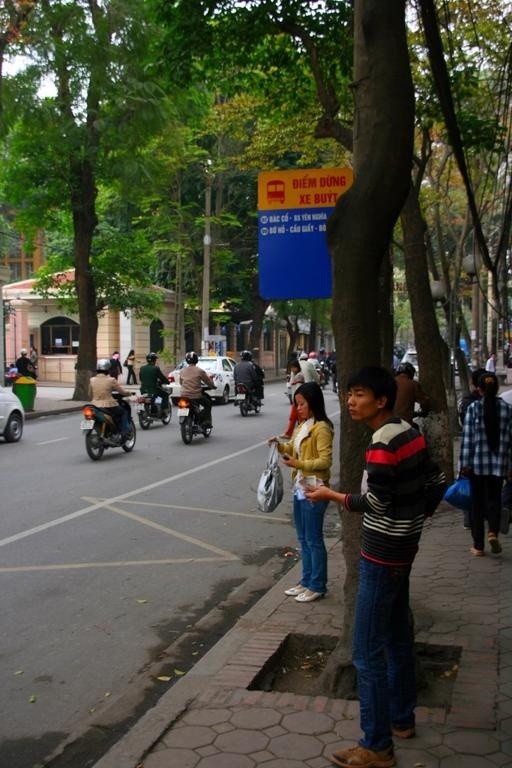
[198,158,216,357]
[426,254,481,342]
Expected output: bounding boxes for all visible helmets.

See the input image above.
[240,349,253,360]
[300,352,308,360]
[185,352,198,365]
[146,352,159,362]
[308,351,317,358]
[319,348,326,353]
[96,359,113,371]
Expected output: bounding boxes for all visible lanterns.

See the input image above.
[462,253,480,284]
[431,281,448,306]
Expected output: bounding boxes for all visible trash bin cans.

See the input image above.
[14,376,39,412]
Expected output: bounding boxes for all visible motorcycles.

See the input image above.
[234,353,338,416]
[80,389,139,462]
[168,385,219,445]
[134,377,173,430]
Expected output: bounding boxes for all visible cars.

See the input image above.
[165,355,240,407]
[0,383,26,442]
[392,341,460,381]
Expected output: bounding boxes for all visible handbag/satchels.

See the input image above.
[256,442,284,513]
[123,360,127,366]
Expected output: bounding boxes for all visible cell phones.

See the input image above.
[282,454,290,461]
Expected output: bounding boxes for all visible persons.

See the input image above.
[139,352,171,418]
[9,346,38,386]
[126,350,138,384]
[110,352,122,379]
[207,343,218,356]
[179,351,215,428]
[88,359,132,437]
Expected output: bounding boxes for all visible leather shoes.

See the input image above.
[294,589,329,602]
[284,583,308,596]
[329,743,398,768]
[393,718,417,738]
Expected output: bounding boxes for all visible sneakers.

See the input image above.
[487,532,502,554]
[468,542,485,556]
